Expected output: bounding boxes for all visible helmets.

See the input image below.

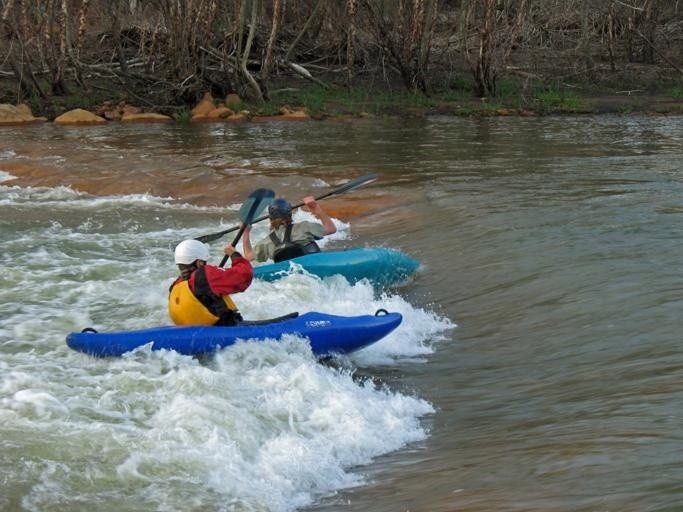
[268,198,292,221]
[174,239,211,266]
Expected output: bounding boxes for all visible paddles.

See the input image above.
[220,189,274,269]
[169,171,380,251]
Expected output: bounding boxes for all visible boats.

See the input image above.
[65,308,403,363]
[253,247,420,290]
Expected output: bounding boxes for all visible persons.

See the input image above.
[167,239,254,328]
[239,196,338,264]
